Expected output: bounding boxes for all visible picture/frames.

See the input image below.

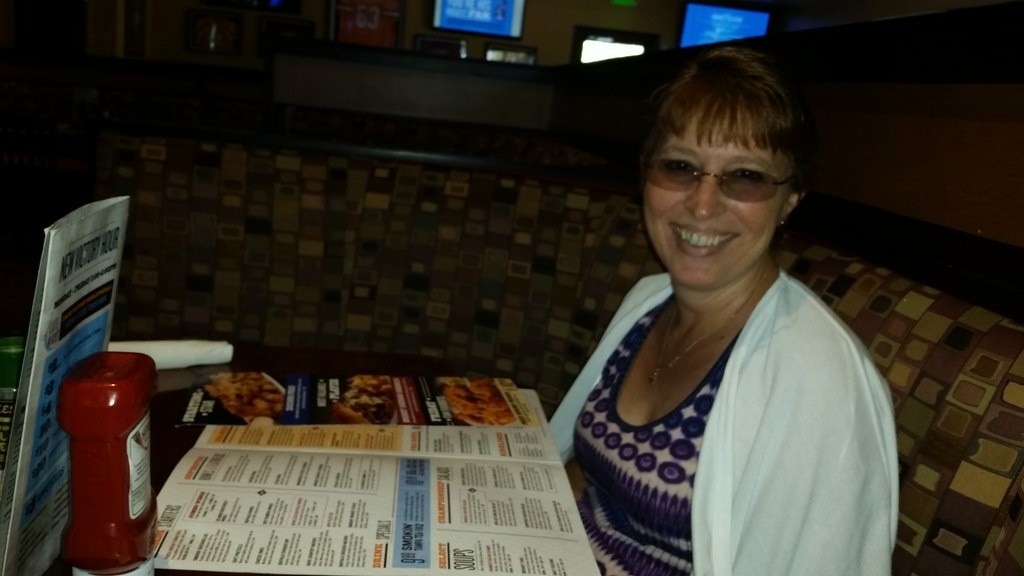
[323,0,407,49]
[184,7,245,57]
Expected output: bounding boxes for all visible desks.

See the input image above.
[0,325,602,576]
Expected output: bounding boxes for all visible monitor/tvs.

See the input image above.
[568,25,661,65]
[674,0,776,51]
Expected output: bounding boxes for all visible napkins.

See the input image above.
[107,340,234,370]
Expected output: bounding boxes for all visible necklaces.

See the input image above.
[645,308,739,388]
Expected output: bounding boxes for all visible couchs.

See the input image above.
[89,106,1024,576]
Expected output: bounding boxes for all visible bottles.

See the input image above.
[55,351,157,576]
[0,337,26,494]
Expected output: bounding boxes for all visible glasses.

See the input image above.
[642,155,790,201]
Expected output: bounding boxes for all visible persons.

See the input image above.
[546,53,899,576]
